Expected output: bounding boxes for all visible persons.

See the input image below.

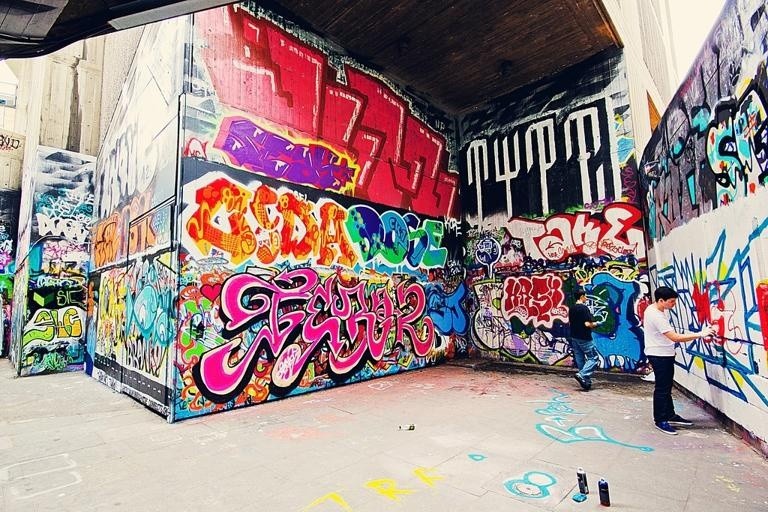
[569,289,597,389]
[644,287,716,435]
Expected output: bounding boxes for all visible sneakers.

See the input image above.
[654,415,693,434]
[575,373,591,388]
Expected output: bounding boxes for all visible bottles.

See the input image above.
[592,322,599,327]
[396,423,416,431]
[704,327,713,342]
[577,466,590,495]
[598,477,611,506]
